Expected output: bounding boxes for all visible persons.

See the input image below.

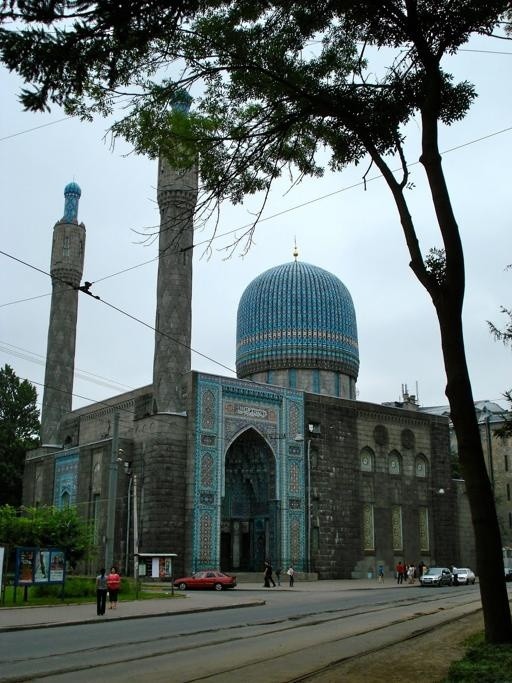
[449,562,460,586]
[107,566,121,609]
[275,566,283,580]
[287,564,294,586]
[96,568,108,616]
[264,560,276,586]
[378,560,428,584]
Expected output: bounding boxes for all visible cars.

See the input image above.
[173,570,238,591]
[420,567,454,587]
[451,568,476,585]
